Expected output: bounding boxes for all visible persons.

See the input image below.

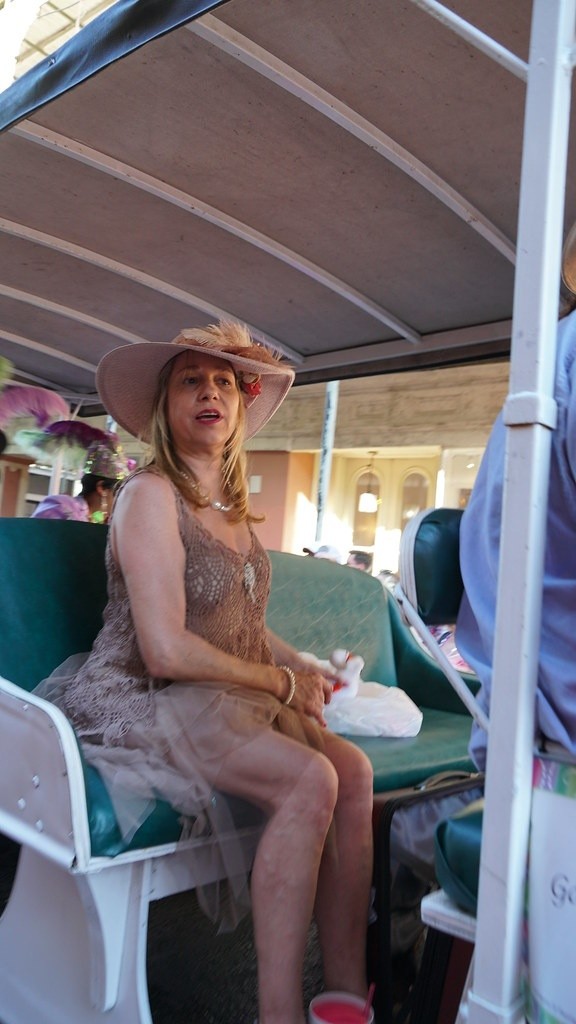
[456,307,576,775]
[19,321,373,1024]
[345,547,372,573]
[29,444,131,525]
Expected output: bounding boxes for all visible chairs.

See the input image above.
[397,503,464,628]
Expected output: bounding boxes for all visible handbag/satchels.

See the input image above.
[299,649,423,739]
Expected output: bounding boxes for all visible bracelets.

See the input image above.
[276,665,296,706]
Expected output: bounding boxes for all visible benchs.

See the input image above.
[1,516,495,1022]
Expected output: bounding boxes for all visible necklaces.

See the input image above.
[174,465,236,513]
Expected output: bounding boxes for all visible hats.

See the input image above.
[0,384,133,481]
[95,317,296,451]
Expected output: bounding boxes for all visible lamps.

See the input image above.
[357,450,381,514]
